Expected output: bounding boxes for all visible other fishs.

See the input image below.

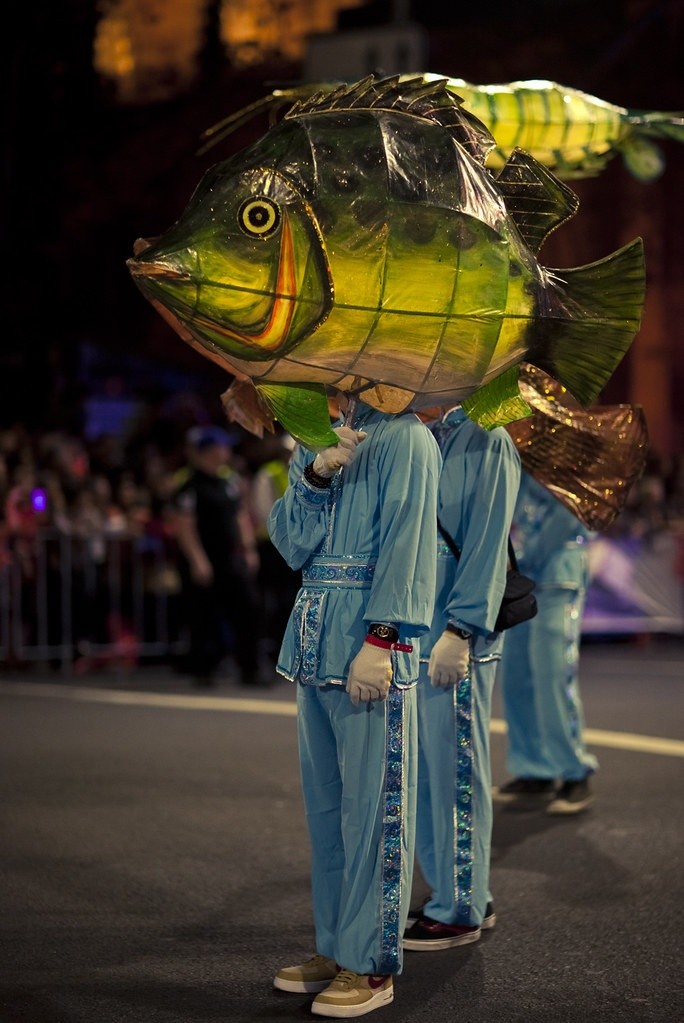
[124,70,684,531]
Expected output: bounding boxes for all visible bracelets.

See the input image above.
[365,634,413,653]
[304,461,331,488]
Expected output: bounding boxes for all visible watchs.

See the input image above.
[446,623,472,639]
[368,623,399,642]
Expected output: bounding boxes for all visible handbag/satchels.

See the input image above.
[495,570,537,631]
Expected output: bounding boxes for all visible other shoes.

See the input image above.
[494,777,554,802]
[547,779,592,813]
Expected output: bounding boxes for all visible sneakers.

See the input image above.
[311,967,394,1018]
[273,954,342,993]
[483,902,497,930]
[402,914,482,953]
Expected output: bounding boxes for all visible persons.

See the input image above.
[265,379,603,1019]
[0,421,301,690]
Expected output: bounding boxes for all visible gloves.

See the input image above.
[313,426,367,480]
[346,640,393,707]
[428,630,469,687]
